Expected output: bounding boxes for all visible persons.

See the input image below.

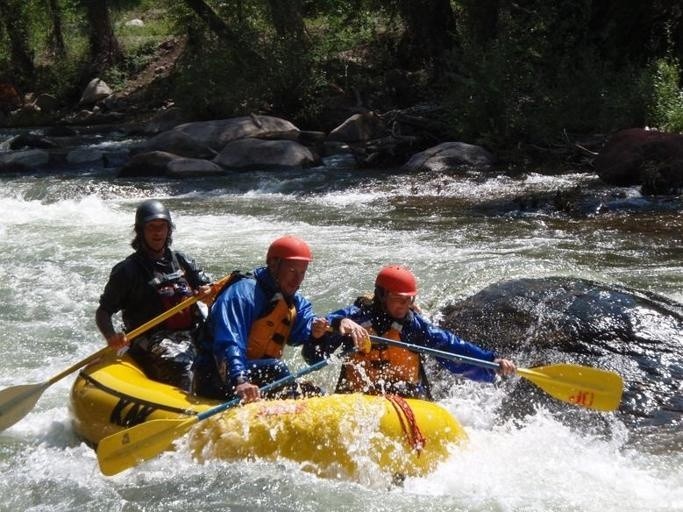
[190,234,329,406]
[93,199,215,394]
[299,266,516,403]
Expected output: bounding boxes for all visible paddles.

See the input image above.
[96,338,372,476]
[364,335,624,412]
[0,294,202,432]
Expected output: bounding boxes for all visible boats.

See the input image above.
[68,350,472,493]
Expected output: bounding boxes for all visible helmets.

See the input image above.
[265,236,311,262]
[135,199,171,225]
[374,265,416,297]
